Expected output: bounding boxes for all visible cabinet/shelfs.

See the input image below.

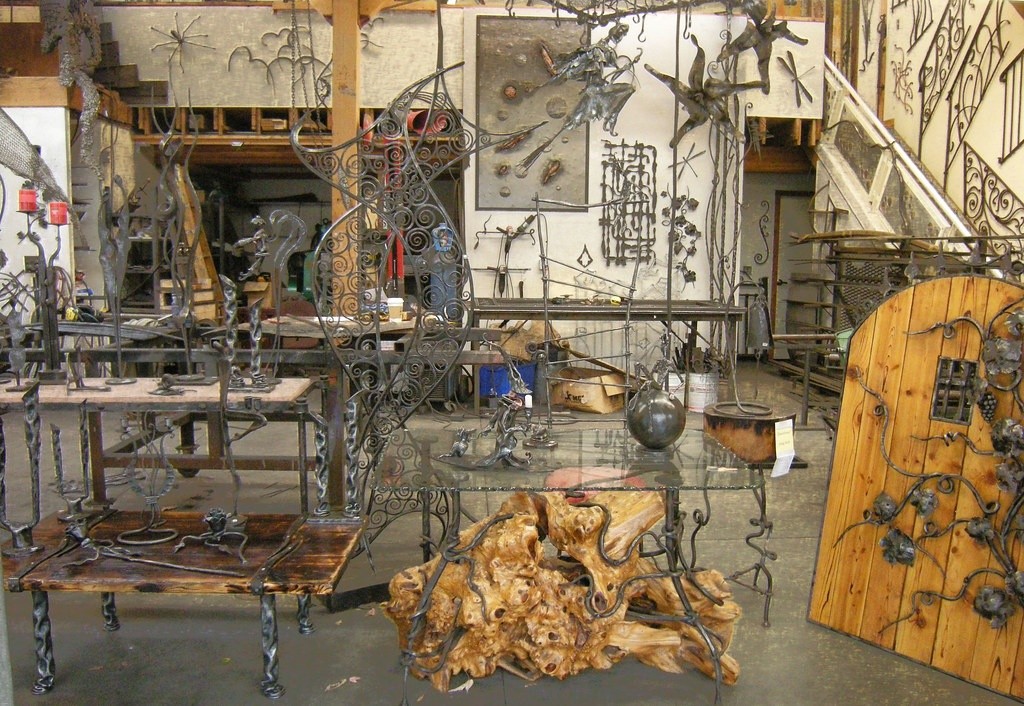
[0,377,368,698]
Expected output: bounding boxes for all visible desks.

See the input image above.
[464,295,745,418]
[363,427,808,706]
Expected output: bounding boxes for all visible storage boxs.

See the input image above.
[242,279,276,310]
[477,362,537,404]
[543,365,626,414]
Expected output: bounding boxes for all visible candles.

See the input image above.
[48,201,68,225]
[19,189,37,212]
